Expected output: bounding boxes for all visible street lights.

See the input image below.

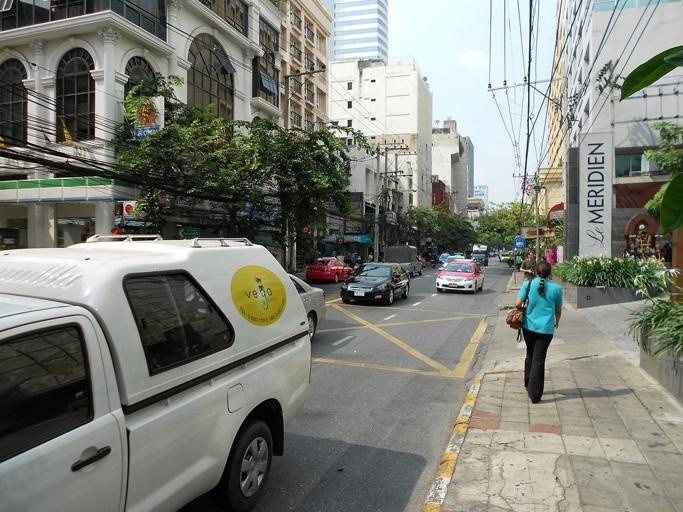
[398,189,417,196]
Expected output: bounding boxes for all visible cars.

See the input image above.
[489,250,510,262]
[288,257,410,340]
[436,253,484,294]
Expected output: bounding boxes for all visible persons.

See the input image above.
[519,250,537,281]
[515,260,564,405]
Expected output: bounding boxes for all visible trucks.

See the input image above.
[471,244,488,266]
[384,245,423,278]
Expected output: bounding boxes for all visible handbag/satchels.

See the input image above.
[505,304,528,330]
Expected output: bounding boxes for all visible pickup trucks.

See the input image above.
[0,234,311,512]
